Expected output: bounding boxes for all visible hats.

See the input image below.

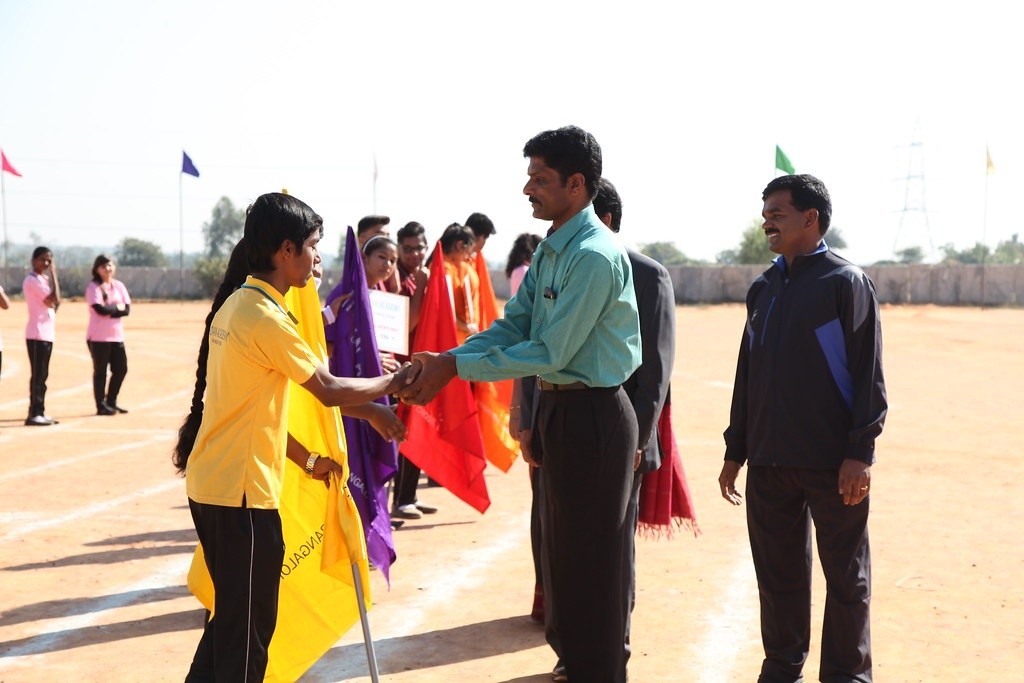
[358,216,390,232]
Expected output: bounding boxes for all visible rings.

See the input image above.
[860,486,869,491]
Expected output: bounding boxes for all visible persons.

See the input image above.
[86,254,131,415]
[392,126,643,683]
[23,246,61,425]
[175,178,673,623]
[185,191,422,683]
[0,284,9,379]
[718,173,887,683]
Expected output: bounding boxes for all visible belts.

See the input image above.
[535,376,592,391]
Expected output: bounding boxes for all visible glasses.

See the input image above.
[400,242,426,252]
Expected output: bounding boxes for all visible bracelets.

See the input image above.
[306,451,322,476]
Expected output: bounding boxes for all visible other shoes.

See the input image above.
[111,406,127,413]
[97,402,114,414]
[551,663,568,680]
[391,503,422,518]
[529,613,545,623]
[25,415,58,425]
[415,501,437,512]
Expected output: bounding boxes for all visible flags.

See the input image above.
[775,147,797,176]
[184,154,199,177]
[986,152,994,174]
[1,150,22,177]
[186,225,699,683]
